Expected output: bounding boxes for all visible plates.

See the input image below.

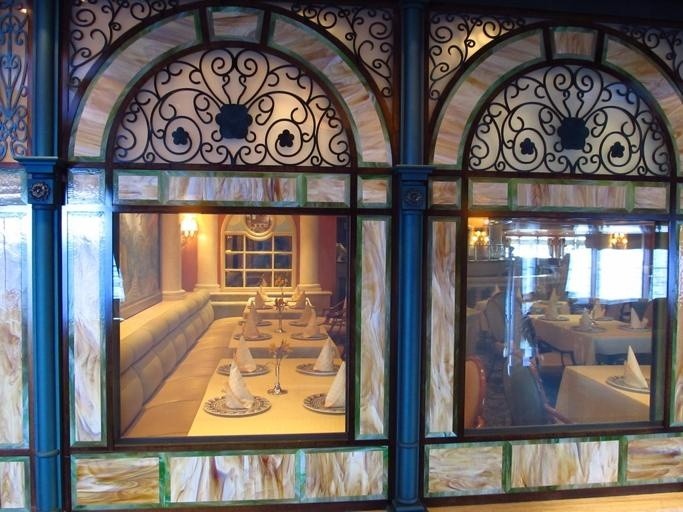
[203,296,351,416]
[605,375,655,394]
[536,312,652,334]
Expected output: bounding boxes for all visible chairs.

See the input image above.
[463,288,666,429]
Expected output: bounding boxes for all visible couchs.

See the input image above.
[120,290,243,439]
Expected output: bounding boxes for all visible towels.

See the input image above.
[254,284,306,307]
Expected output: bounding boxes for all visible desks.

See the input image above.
[187,296,345,437]
